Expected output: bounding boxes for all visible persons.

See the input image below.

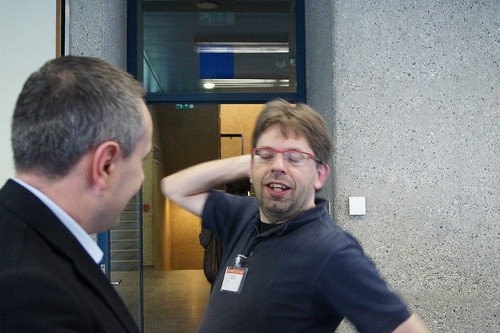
[0,54,155,333]
[198,174,252,285]
[160,98,430,333]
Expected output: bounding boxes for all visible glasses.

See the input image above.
[251,146,322,166]
